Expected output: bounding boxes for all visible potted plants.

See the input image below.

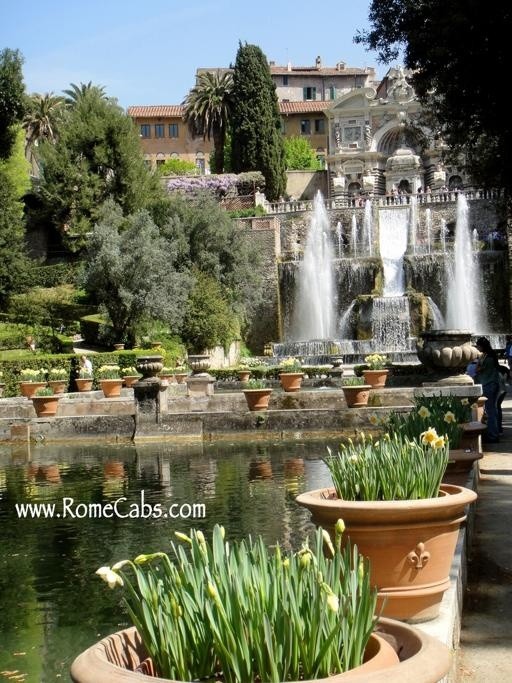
[32,389,60,416]
[242,378,371,411]
[25,458,63,502]
[70,392,484,682]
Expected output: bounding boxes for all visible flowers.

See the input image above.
[16,355,390,378]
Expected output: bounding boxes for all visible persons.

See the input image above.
[467,333,512,443]
[273,181,491,211]
[75,355,93,379]
[478,226,508,251]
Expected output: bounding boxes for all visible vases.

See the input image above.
[16,370,389,401]
